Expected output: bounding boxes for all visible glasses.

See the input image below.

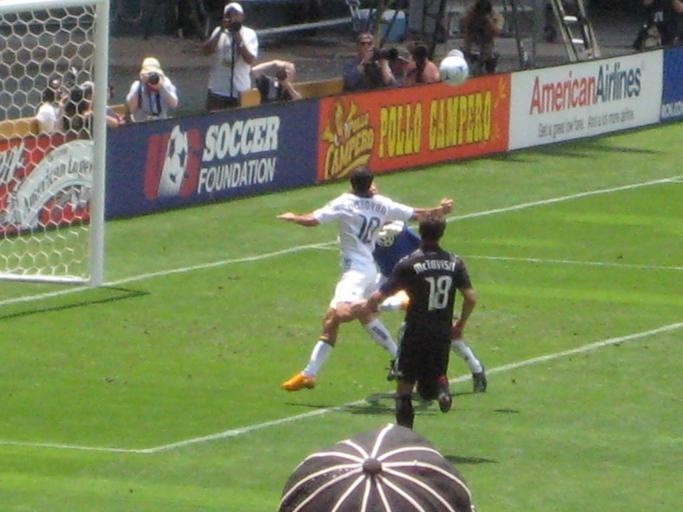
[359,40,374,47]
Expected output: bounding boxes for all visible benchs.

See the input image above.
[238,75,343,108]
[0,104,128,141]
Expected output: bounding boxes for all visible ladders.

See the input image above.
[551,0,600,63]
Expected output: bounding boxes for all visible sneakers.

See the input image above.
[438,374,452,414]
[281,372,316,390]
[472,365,488,394]
[386,355,398,382]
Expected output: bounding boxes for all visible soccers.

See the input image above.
[439,56,469,85]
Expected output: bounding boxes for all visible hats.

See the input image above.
[223,2,245,17]
[139,56,164,75]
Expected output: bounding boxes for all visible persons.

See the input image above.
[277,169,455,392]
[372,217,488,396]
[343,4,506,94]
[645,0,683,45]
[367,210,476,431]
[30,3,301,136]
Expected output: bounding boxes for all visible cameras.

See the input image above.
[475,2,491,15]
[373,47,398,60]
[147,73,158,84]
[276,68,289,80]
[228,17,241,32]
